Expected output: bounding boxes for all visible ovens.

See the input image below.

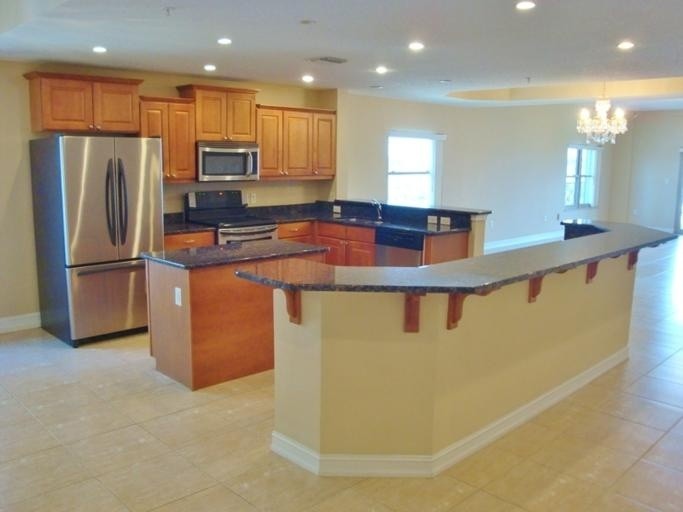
[217,225,277,246]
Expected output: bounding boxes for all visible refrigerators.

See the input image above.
[27,135,167,350]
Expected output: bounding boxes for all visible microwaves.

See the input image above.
[196,141,260,182]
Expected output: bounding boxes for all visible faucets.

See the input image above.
[371,197,383,219]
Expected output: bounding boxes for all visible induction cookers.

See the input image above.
[186,215,279,233]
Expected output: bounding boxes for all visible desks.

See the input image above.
[139,238,332,391]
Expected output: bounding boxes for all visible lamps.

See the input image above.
[576,81,629,147]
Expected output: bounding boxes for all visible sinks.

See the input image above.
[335,217,383,227]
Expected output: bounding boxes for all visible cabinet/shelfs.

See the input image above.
[163,231,216,251]
[315,219,376,266]
[29,77,143,134]
[138,96,196,183]
[255,103,337,182]
[278,220,315,244]
[179,90,256,142]
